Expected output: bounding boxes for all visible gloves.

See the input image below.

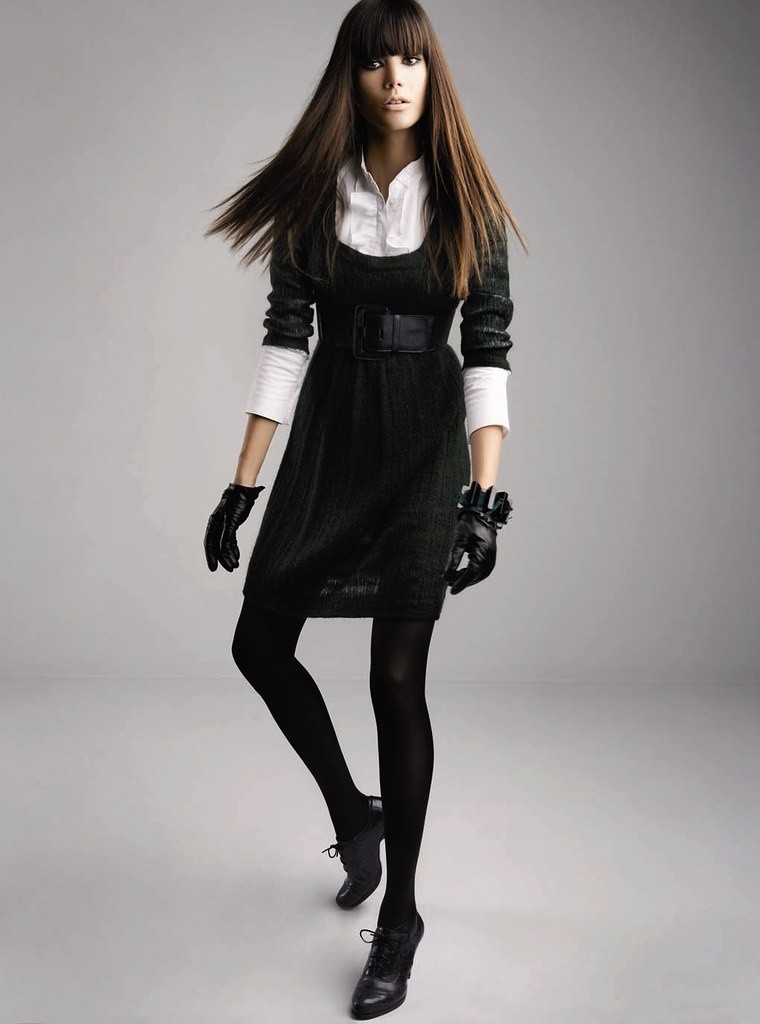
[203,484,265,572]
[444,481,512,594]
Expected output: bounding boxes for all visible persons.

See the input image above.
[203,0,531,1021]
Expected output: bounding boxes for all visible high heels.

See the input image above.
[350,911,424,1019]
[322,794,384,909]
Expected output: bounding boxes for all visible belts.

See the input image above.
[317,303,454,360]
